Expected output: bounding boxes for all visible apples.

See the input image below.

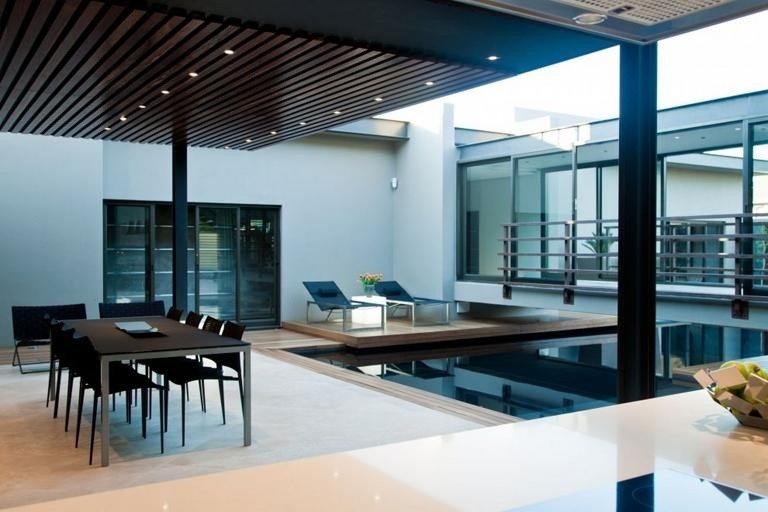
[714,360,768,417]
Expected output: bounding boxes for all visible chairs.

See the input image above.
[44,307,252,467]
[301,279,453,332]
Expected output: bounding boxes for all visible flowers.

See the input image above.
[357,272,383,286]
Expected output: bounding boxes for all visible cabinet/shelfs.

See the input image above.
[102,198,282,331]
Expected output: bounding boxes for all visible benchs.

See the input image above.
[11,299,165,375]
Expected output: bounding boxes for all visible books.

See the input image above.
[113,321,160,333]
